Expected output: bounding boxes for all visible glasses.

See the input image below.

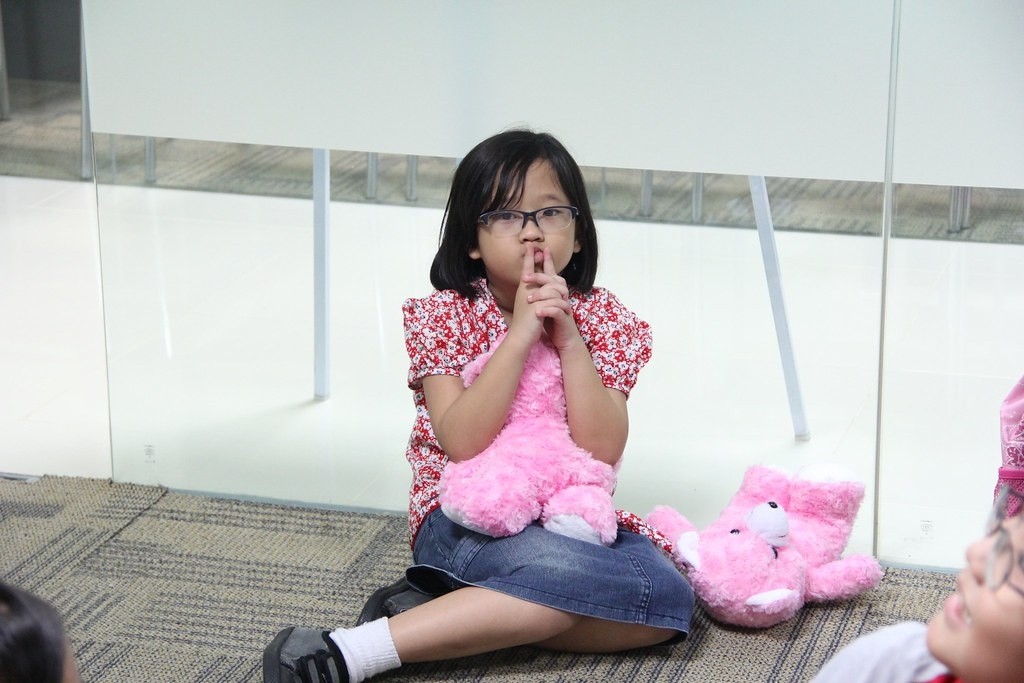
[984,485,1024,597]
[477,205,578,237]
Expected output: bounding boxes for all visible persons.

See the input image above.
[800,513,1024,683]
[264,133,694,683]
[0,585,82,683]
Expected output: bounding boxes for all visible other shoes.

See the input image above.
[262,627,350,683]
[355,576,435,627]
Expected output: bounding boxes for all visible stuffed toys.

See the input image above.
[645,467,881,627]
[440,330,618,544]
